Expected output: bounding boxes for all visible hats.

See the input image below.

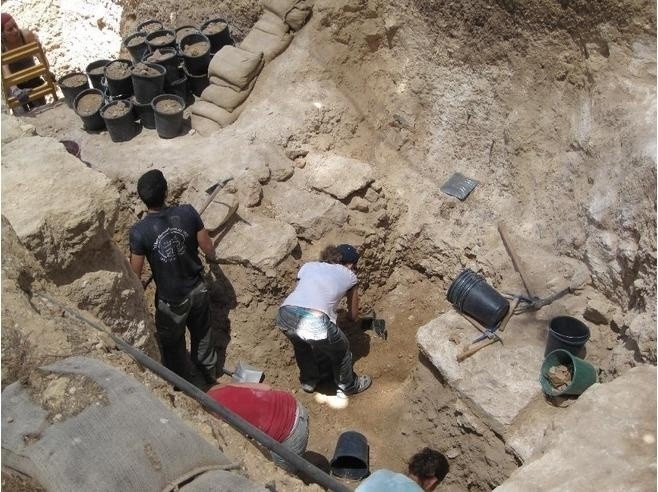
[337,244,359,272]
[1,13,11,25]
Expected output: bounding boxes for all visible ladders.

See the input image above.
[2,40,58,115]
[220,361,266,386]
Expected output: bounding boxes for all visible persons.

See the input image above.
[127,169,219,393]
[353,448,448,492]
[277,243,370,397]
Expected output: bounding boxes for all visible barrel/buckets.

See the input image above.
[539,349,598,397]
[329,429,370,480]
[445,269,510,329]
[58,16,232,157]
[545,316,591,360]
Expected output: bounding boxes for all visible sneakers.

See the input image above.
[302,367,328,392]
[336,375,371,398]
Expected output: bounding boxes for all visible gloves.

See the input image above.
[12,87,32,105]
[42,71,55,81]
